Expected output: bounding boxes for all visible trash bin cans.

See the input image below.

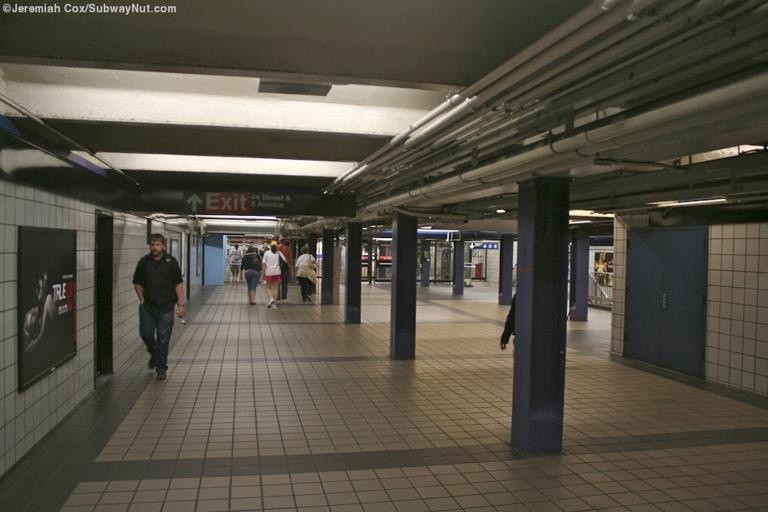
[475,262,483,279]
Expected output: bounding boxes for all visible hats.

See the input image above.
[270,240,278,246]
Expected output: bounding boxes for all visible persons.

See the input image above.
[228,239,319,310]
[500,295,516,350]
[21,266,58,355]
[132,234,186,380]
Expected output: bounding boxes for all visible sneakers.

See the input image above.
[303,295,311,301]
[266,299,289,310]
[148,358,168,380]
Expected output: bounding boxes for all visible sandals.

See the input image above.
[249,299,256,305]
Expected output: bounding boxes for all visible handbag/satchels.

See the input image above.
[307,270,317,284]
[252,258,262,270]
[279,256,285,269]
